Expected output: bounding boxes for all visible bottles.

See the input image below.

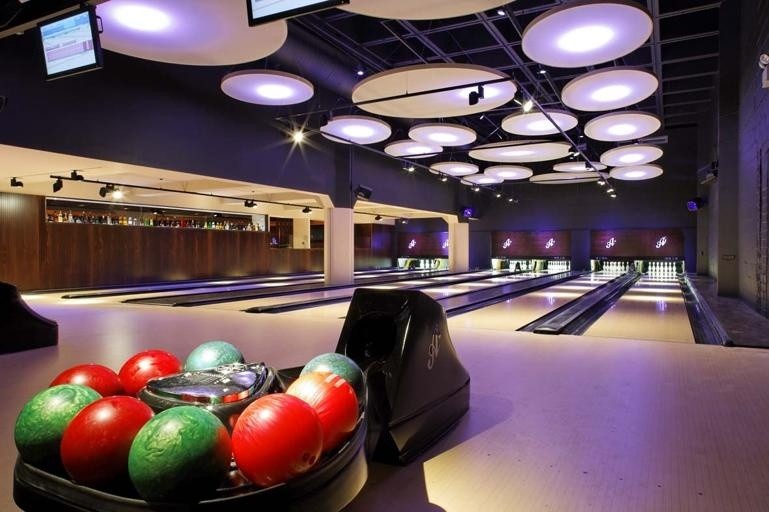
[52,209,260,231]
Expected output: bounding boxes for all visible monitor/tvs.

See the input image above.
[37,6,104,85]
[247,0,350,27]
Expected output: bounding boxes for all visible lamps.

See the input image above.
[219,69,314,106]
[51,171,396,221]
[384,139,443,159]
[95,1,287,67]
[319,116,391,145]
[11,177,23,187]
[561,66,659,113]
[408,123,476,147]
[537,63,546,74]
[350,64,517,119]
[600,144,663,166]
[399,161,664,202]
[759,54,769,88]
[521,1,654,68]
[468,140,573,163]
[336,1,514,21]
[584,111,661,142]
[501,109,579,136]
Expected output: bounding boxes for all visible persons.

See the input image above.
[271,236,278,244]
